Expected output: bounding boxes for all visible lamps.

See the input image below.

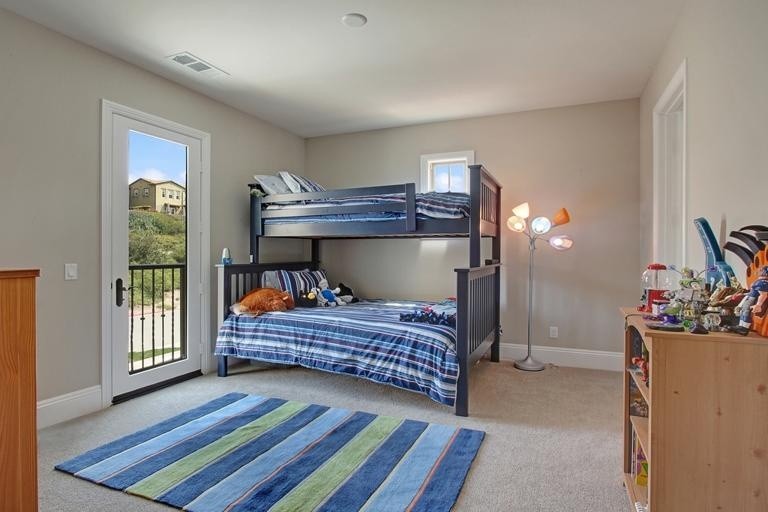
[507,200,576,372]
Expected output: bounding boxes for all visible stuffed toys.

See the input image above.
[298,279,359,308]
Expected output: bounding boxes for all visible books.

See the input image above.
[630,424,648,487]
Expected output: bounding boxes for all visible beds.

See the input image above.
[215,158,506,418]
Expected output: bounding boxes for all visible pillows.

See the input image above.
[253,169,331,202]
[264,265,328,304]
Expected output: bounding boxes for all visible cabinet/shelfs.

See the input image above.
[615,304,768,511]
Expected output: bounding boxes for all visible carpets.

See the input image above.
[52,377,490,510]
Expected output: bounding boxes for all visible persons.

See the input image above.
[728,267,768,336]
